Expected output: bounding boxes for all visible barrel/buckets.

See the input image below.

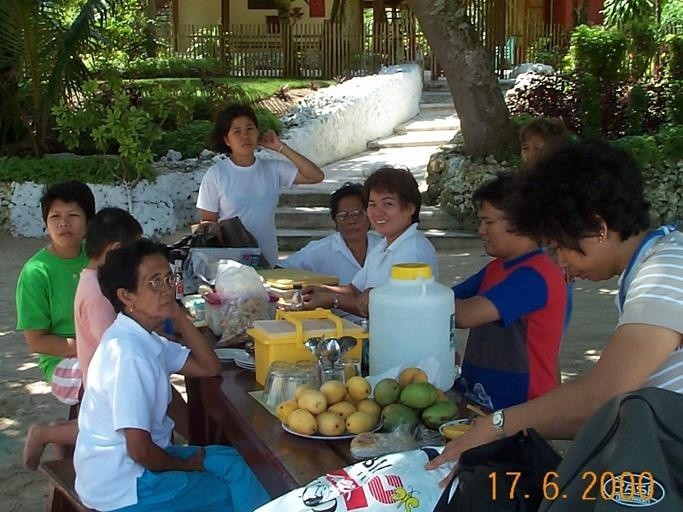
[368,263,457,393]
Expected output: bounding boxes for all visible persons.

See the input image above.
[449,176,567,416]
[299,165,440,318]
[196,100,324,269]
[16,177,96,405]
[519,117,573,330]
[427,137,683,488]
[23,207,145,472]
[73,235,273,511]
[273,181,385,326]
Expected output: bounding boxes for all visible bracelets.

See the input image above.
[334,293,339,309]
[277,143,286,153]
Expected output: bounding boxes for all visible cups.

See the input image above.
[262,358,361,410]
[189,224,199,234]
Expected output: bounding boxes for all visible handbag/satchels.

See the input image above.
[168,216,272,268]
[539,388,683,512]
[434,428,562,512]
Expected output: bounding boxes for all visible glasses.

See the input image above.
[335,209,366,224]
[141,273,180,288]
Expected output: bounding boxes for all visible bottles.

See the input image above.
[290,285,304,311]
[331,294,342,316]
[173,260,184,303]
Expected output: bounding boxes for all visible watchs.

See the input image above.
[491,409,507,439]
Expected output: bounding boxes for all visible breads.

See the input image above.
[442,424,471,440]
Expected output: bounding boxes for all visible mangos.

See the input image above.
[275,366,459,436]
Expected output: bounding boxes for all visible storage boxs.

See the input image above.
[243,303,367,386]
[252,267,341,313]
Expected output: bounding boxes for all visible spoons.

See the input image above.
[304,337,356,366]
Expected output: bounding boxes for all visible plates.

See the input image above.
[438,419,476,438]
[213,348,255,371]
[281,422,383,440]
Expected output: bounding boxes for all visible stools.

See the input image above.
[35,457,97,512]
